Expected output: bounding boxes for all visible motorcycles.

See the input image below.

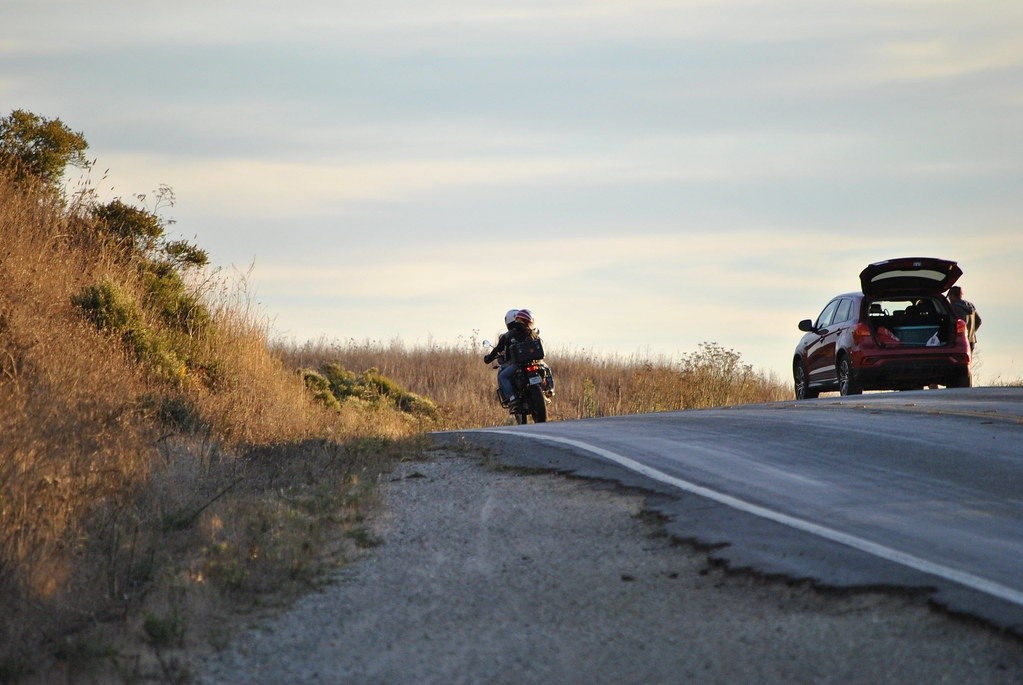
[484,325,553,426]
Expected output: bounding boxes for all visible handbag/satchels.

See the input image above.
[925,331,941,348]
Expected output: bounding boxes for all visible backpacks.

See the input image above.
[505,331,545,362]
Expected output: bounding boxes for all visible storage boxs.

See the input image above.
[891,326,942,348]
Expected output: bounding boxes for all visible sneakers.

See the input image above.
[507,399,518,406]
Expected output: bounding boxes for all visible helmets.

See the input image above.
[513,309,534,324]
[505,308,520,325]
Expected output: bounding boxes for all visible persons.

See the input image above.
[484,308,553,402]
[949,285,982,351]
[499,310,554,404]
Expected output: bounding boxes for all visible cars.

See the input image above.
[793,255,974,398]
